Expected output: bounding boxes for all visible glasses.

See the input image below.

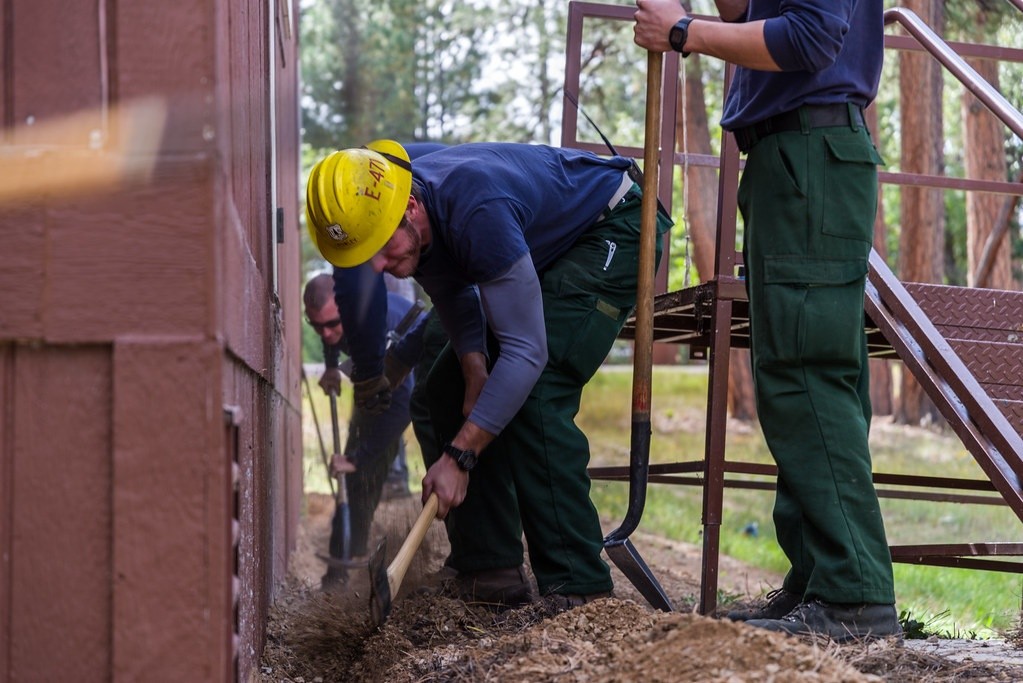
[305,318,340,328]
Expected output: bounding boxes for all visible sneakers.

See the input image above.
[745,598,906,644]
[725,588,804,622]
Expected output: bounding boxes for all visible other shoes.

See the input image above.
[407,566,534,614]
[497,591,617,627]
[321,570,350,592]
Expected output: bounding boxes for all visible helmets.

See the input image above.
[305,140,413,268]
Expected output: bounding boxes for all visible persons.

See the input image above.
[633,0,898,644]
[302,139,675,612]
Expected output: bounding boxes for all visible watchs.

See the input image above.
[444,444,478,471]
[668,17,691,58]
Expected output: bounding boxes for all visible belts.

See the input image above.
[733,104,864,151]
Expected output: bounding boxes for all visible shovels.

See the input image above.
[315,386,373,593]
